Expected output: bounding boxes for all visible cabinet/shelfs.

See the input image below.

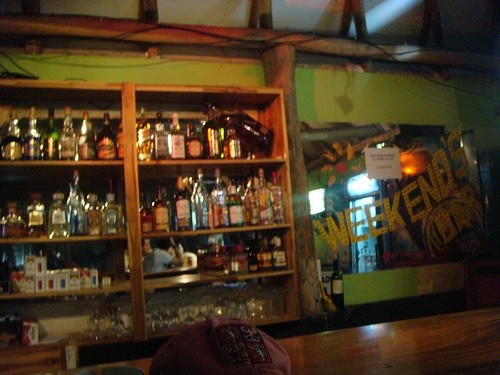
[0,79,300,354]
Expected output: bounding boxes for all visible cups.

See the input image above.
[86,285,275,340]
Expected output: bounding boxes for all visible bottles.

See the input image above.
[0,106,274,162]
[0,234,287,293]
[330,259,344,308]
[0,167,286,239]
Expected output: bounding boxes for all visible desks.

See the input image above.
[59,306,500,375]
